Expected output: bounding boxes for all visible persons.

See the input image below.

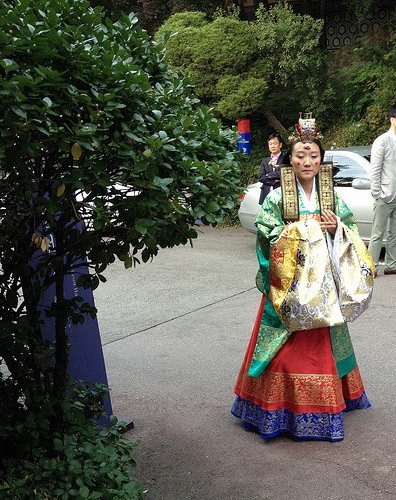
[258,134,289,206]
[231,112,376,442]
[367,104,396,276]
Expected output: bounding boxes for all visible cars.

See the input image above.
[237,146,396,250]
[75,182,193,232]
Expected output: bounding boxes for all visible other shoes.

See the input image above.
[384,268,396,275]
